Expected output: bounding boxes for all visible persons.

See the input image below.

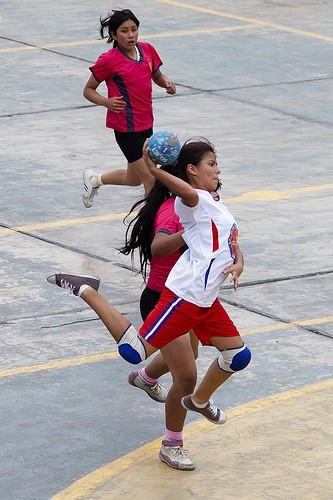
[47,138,252,425]
[82,9,176,208]
[115,162,200,471]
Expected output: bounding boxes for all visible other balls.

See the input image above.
[147,130,181,165]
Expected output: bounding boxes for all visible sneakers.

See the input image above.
[47,272,101,297]
[181,394,228,425]
[127,369,170,403]
[82,169,100,207]
[158,437,196,471]
